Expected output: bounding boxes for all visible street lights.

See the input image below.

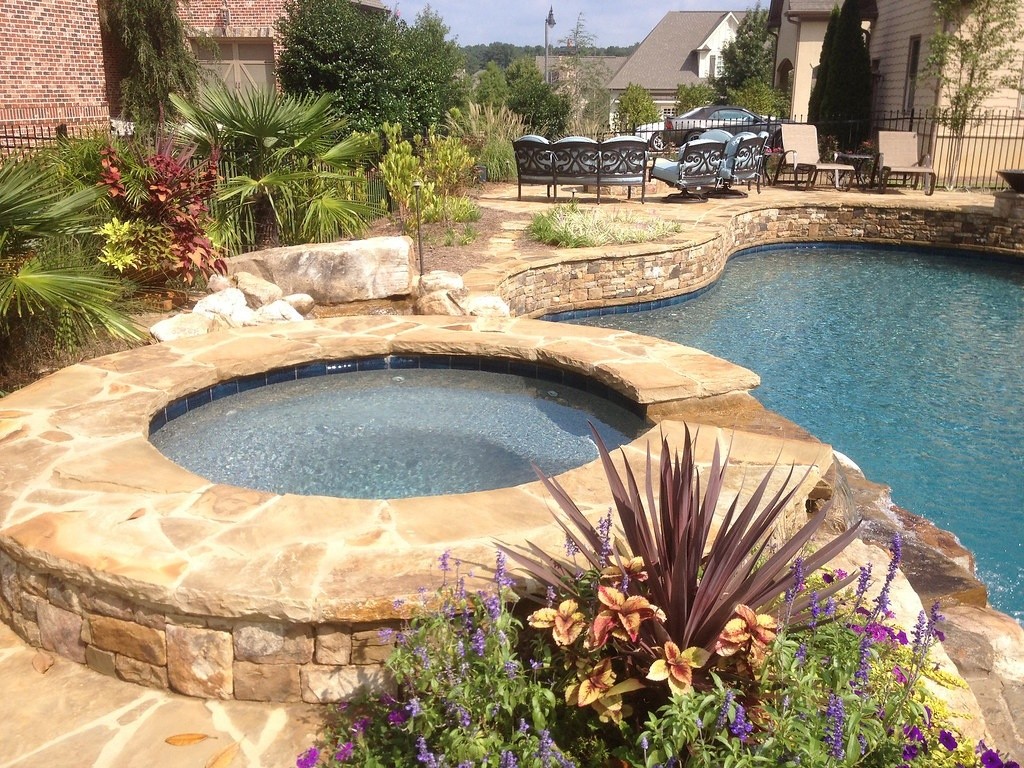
[543,4,556,84]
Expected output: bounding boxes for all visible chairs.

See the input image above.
[650,129,768,204]
[773,124,856,190]
[868,131,936,196]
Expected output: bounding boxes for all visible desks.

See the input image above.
[838,153,873,189]
[762,153,772,186]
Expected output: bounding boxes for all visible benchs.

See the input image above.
[510,133,651,204]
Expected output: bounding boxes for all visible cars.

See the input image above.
[663,105,797,150]
[634,104,775,153]
